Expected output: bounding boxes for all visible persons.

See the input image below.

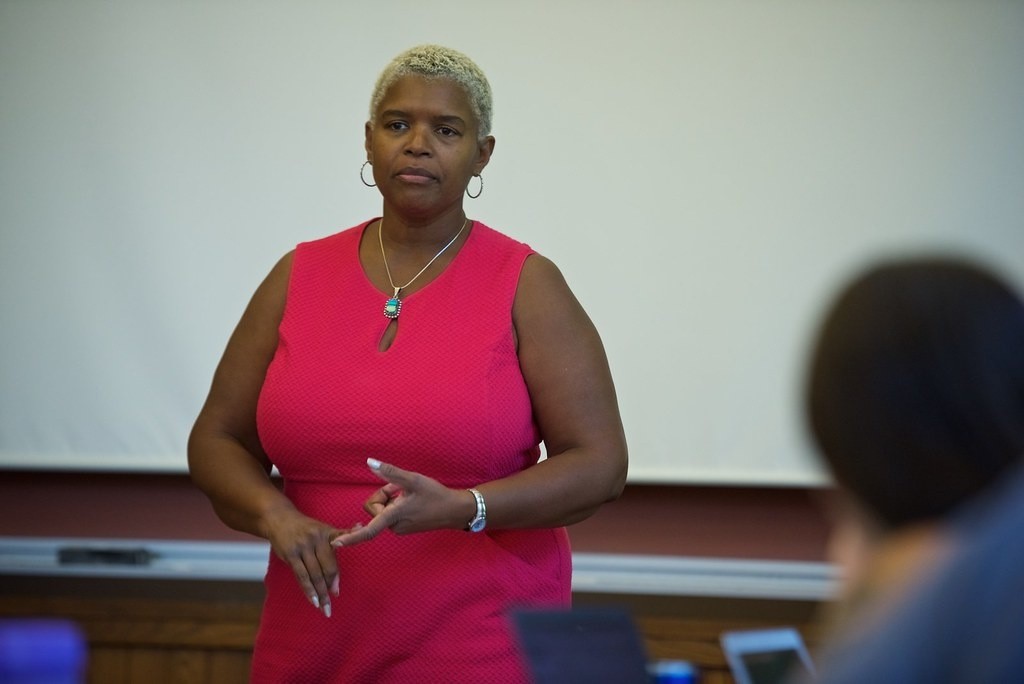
[807,258,1024,684]
[187,45,629,684]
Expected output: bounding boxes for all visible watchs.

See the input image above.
[466,488,486,532]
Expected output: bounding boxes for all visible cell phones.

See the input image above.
[722,625,815,684]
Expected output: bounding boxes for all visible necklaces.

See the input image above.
[380,217,467,318]
